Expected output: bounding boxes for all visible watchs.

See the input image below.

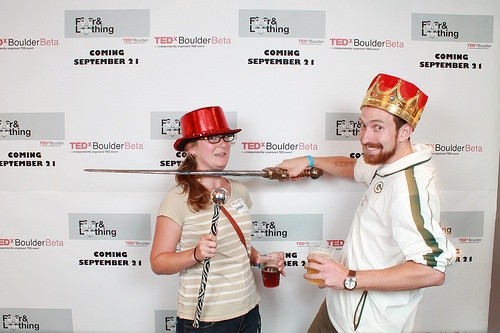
[343,270,357,291]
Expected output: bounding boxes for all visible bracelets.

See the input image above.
[306,155,314,169]
[194,246,202,263]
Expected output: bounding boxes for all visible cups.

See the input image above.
[307,242,336,285]
[257,251,283,288]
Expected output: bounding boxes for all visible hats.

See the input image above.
[174,106,241,152]
[360,73,428,131]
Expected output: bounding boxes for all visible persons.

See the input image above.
[151,106,285,333]
[275,73,457,333]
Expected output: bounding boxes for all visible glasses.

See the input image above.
[191,133,234,143]
